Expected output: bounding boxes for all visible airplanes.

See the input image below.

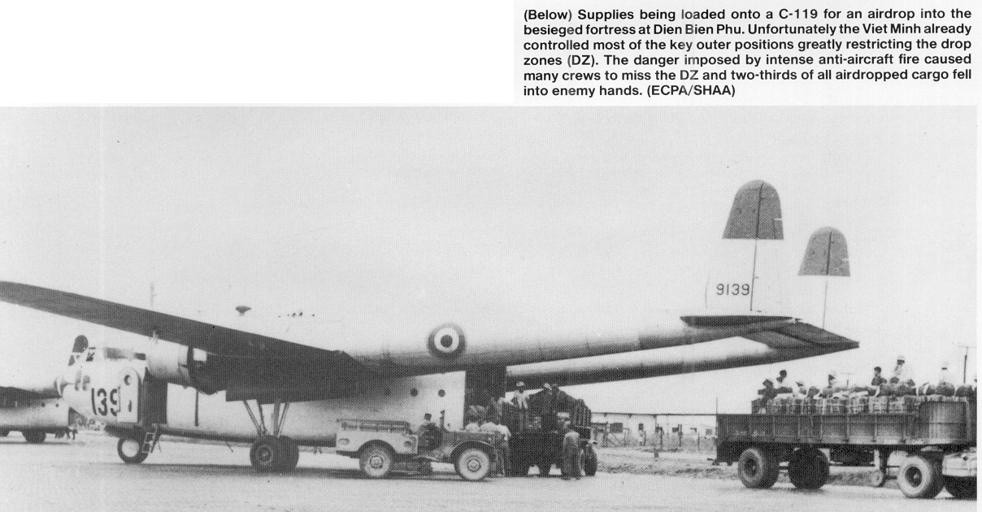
[0,178,859,483]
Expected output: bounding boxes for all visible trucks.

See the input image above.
[714,390,976,499]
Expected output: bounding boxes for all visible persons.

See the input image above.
[415,380,562,476]
[743,354,958,412]
